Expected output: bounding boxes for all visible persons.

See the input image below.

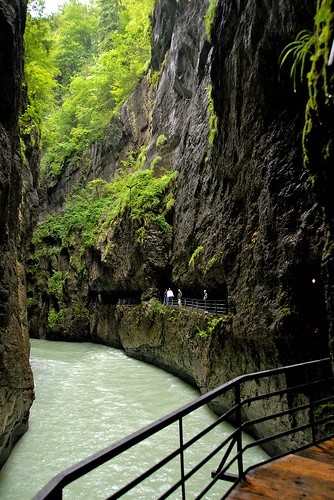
[177,288,182,308]
[166,288,174,306]
[163,289,168,306]
[202,290,208,310]
